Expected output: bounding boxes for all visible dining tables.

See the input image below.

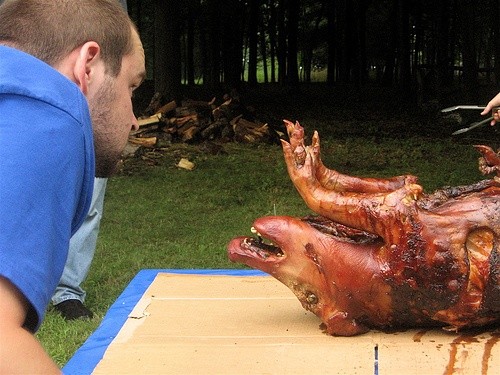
[64,267,499,375]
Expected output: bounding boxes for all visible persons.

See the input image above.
[480,91,500,126]
[2,0,146,374]
[49,0,128,324]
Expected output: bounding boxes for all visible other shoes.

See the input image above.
[55,298,94,321]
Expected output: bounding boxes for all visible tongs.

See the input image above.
[441,105,500,135]
